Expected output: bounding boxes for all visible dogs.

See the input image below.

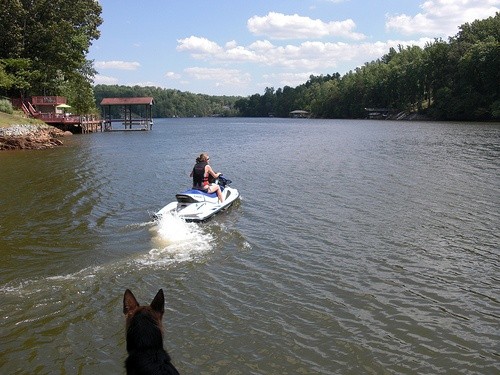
[121,288,179,375]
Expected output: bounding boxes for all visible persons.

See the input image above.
[190,154,224,203]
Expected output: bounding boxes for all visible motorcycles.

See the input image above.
[152,171,240,224]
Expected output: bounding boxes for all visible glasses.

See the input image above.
[207,158,210,160]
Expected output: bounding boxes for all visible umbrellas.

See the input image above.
[56,104,72,114]
